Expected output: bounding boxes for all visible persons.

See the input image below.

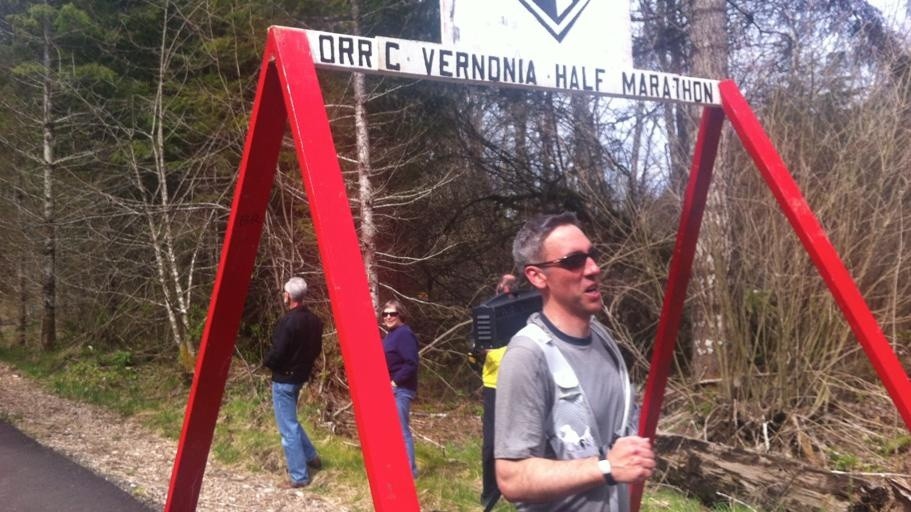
[472,275,524,512]
[263,276,323,487]
[382,298,420,479]
[493,209,657,512]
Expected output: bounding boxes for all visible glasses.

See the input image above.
[523,245,600,274]
[381,310,402,319]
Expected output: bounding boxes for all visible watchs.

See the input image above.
[599,446,614,485]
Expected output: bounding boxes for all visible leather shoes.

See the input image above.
[304,458,322,469]
[276,477,310,488]
